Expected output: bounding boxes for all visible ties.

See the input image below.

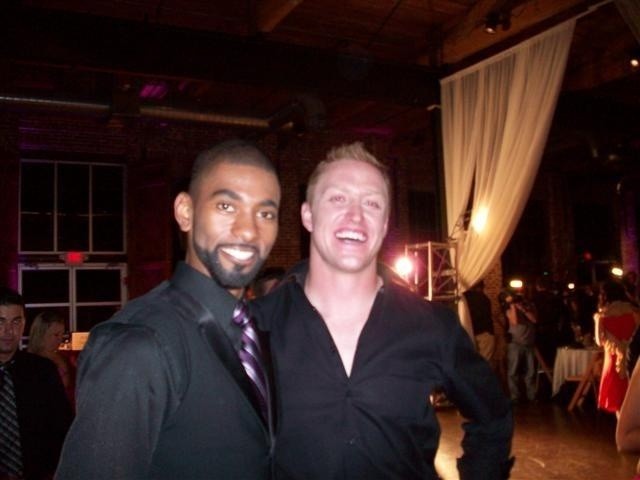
[232,304,273,443]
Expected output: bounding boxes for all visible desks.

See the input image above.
[553,345,602,418]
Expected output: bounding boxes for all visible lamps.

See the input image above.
[483,13,511,34]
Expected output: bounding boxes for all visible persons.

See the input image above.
[22,309,77,407]
[51,138,285,478]
[1,285,75,479]
[461,269,639,479]
[251,143,516,477]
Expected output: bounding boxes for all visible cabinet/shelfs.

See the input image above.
[18,158,127,256]
